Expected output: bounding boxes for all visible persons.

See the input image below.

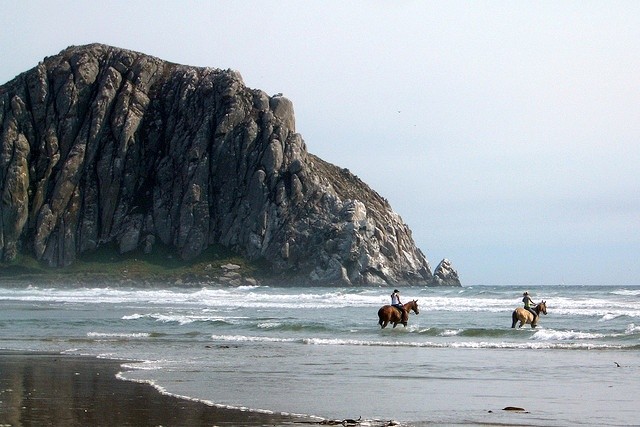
[391,289,407,324]
[522,291,538,326]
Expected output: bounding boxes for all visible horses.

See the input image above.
[511,300,548,330]
[378,299,420,329]
